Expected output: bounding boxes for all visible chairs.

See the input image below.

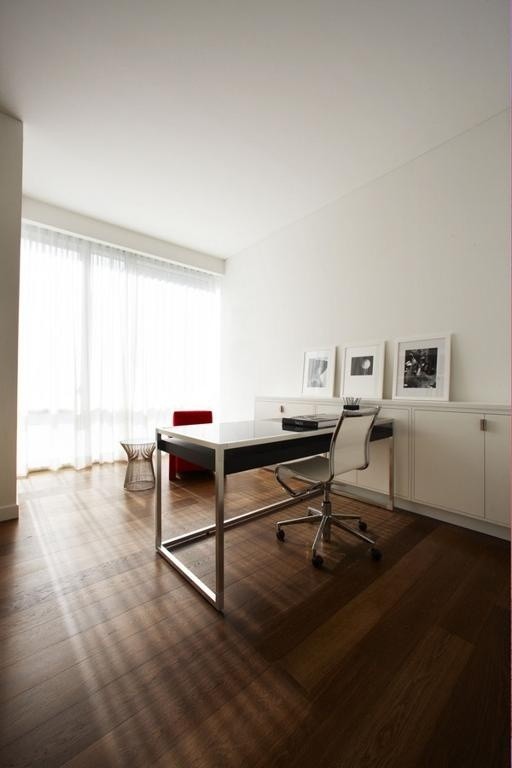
[168,411,213,481]
[275,405,382,568]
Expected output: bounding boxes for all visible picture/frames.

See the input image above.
[300,346,337,399]
[391,334,451,401]
[339,341,385,399]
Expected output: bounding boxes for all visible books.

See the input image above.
[282,413,342,429]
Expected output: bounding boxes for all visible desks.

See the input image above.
[121,442,156,491]
[153,416,395,613]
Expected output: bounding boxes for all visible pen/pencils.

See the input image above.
[343,397,361,405]
